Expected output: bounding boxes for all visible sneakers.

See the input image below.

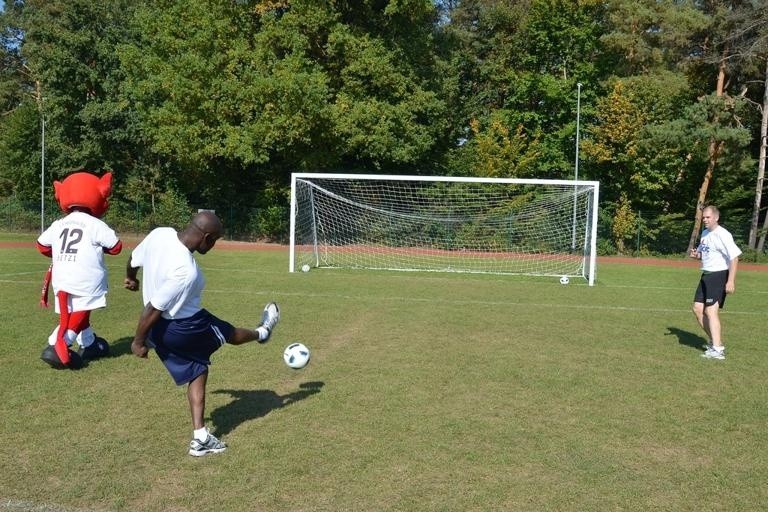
[700,341,726,360]
[188,426,227,457]
[258,301,280,345]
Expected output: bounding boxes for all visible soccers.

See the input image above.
[559,275,569,285]
[282,342,309,369]
[301,264,310,272]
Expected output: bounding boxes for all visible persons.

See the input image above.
[124,211,280,457]
[35,172,123,370]
[689,205,742,362]
